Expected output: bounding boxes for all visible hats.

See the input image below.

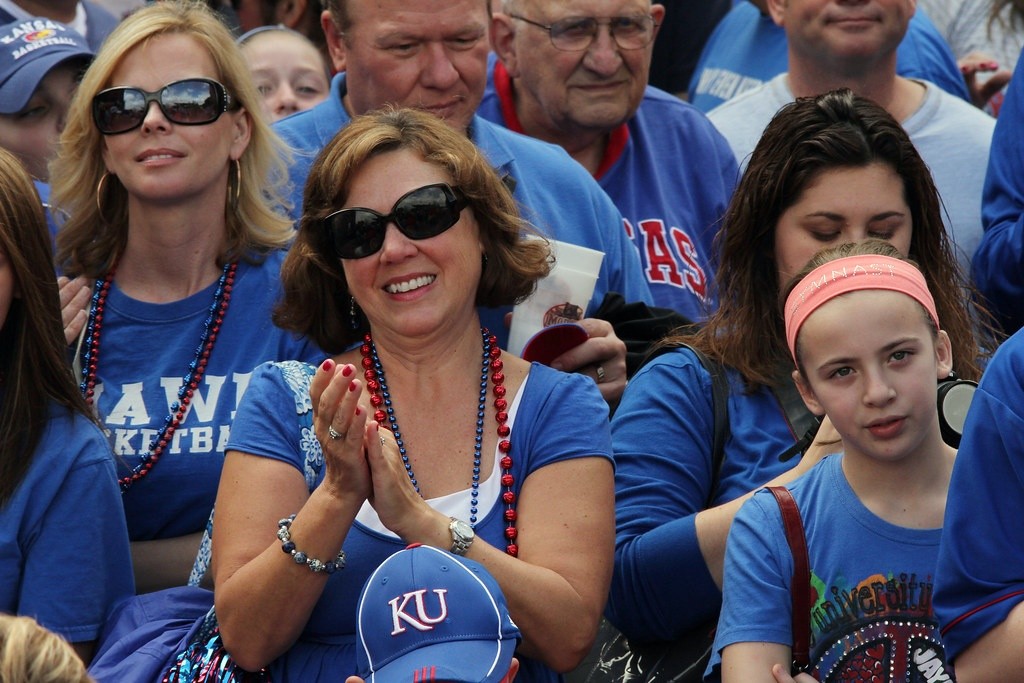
[0,16,97,116]
[357,543,522,683]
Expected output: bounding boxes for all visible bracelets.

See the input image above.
[278,514,346,573]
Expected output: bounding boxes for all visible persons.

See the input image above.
[608,0,1023,683]
[0,0,741,683]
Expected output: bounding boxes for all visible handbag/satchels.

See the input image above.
[561,338,730,683]
[156,605,266,682]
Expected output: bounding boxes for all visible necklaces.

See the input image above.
[80,254,241,496]
[360,327,518,558]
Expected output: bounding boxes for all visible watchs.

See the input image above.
[450,516,475,557]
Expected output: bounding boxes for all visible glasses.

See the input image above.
[90,75,241,135]
[319,181,470,260]
[509,10,657,52]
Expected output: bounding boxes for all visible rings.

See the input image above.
[328,426,343,440]
[379,437,386,446]
[596,365,605,383]
